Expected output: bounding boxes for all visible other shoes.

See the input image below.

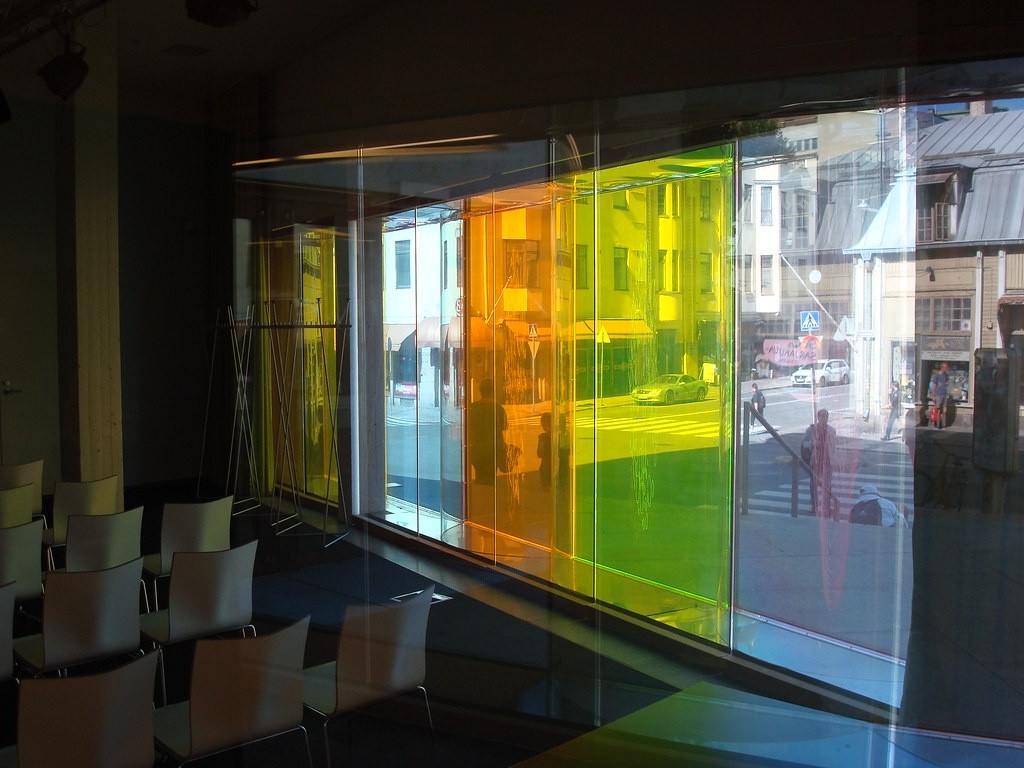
[881,435,890,441]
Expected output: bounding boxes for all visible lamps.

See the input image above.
[925,267,934,276]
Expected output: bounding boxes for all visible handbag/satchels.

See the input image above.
[930,408,937,423]
[801,440,813,467]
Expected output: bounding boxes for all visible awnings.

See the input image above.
[503,317,656,340]
[383,322,417,352]
[414,315,489,350]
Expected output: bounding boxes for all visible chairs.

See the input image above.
[0,459,158,768]
[142,494,235,613]
[140,539,259,707]
[304,584,442,768]
[153,615,314,768]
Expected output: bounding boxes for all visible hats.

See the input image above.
[859,485,882,497]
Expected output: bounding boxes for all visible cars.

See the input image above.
[632,374,708,406]
[395,380,416,395]
[791,359,850,387]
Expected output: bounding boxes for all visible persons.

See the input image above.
[881,381,903,441]
[536,410,553,490]
[931,361,952,429]
[854,484,910,528]
[557,416,572,498]
[802,410,837,516]
[467,379,509,485]
[747,383,767,433]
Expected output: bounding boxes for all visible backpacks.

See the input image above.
[759,395,765,410]
[850,496,883,526]
